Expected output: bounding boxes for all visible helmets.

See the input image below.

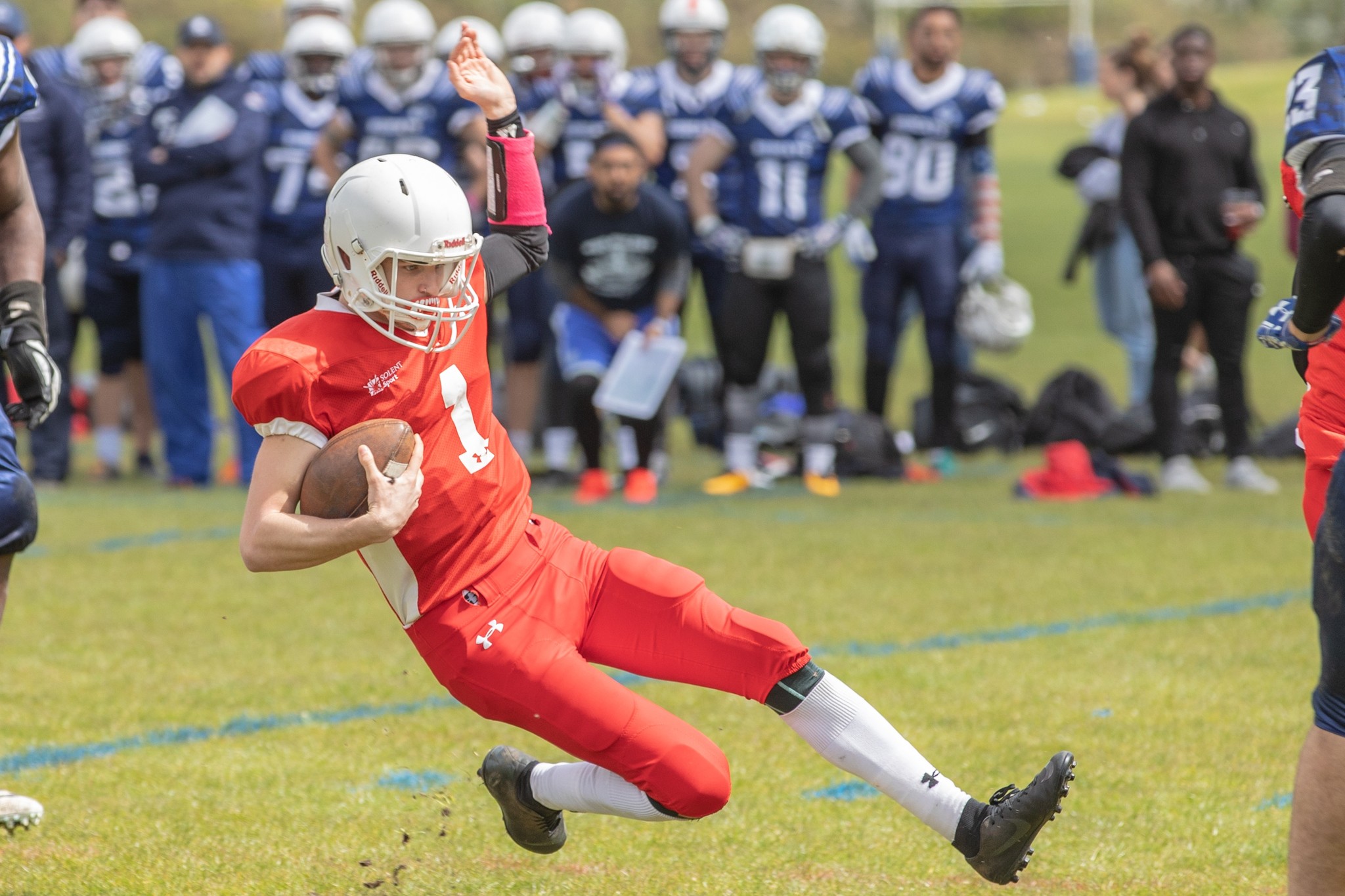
[320,153,472,314]
[652,0,732,36]
[276,1,630,91]
[748,4,828,69]
[952,273,1037,347]
[62,16,146,84]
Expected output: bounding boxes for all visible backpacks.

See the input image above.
[909,366,1306,456]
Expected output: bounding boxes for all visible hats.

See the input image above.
[174,15,228,48]
[0,0,24,38]
[1021,438,1113,501]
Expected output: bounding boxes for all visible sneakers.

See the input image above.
[477,744,567,855]
[962,750,1076,885]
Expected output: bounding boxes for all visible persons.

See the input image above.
[232,25,1077,886]
[839,9,1005,449]
[534,135,695,501]
[128,15,265,489]
[0,0,1345,896]
[686,2,884,496]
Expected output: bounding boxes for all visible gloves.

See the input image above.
[4,339,62,431]
[702,222,752,270]
[1256,295,1342,351]
[791,212,844,264]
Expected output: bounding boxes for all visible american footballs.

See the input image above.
[293,419,412,525]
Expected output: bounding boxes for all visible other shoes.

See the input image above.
[1225,454,1281,495]
[621,467,658,504]
[1158,456,1214,496]
[527,468,571,493]
[0,789,44,836]
[571,467,614,503]
[800,471,841,499]
[700,468,756,496]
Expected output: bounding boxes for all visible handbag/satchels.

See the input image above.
[786,406,905,479]
